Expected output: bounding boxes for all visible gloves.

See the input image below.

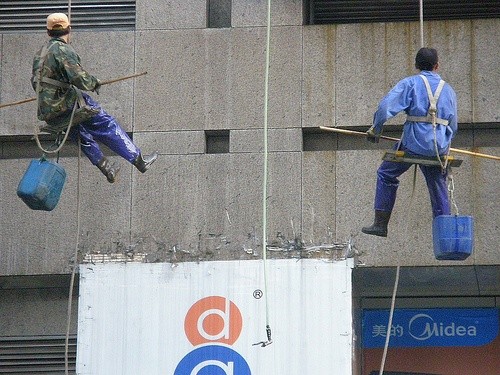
[92,79,102,95]
[365,126,382,144]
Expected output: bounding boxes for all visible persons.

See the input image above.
[31,13,157,183]
[362,46,458,236]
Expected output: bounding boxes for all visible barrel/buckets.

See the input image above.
[431,190,474,261]
[16,143,66,211]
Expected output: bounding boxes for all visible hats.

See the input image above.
[46,12,70,30]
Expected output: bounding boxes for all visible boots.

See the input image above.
[133,153,157,173]
[97,158,121,184]
[362,211,392,238]
